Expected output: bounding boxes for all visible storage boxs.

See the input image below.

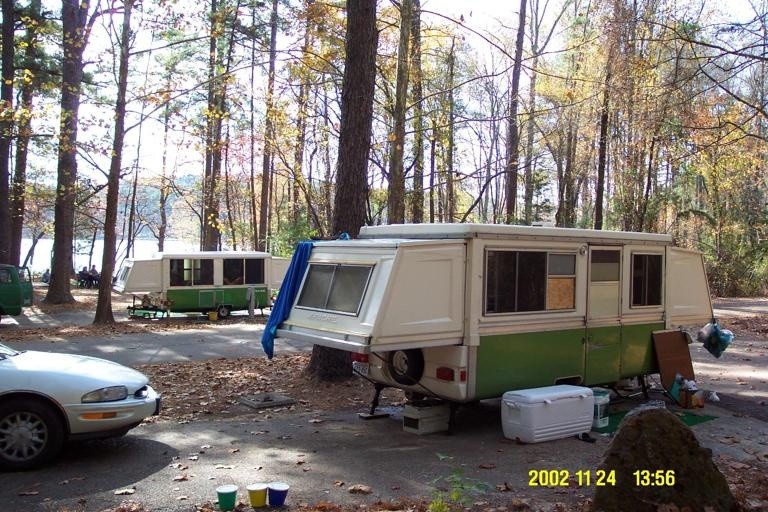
[403,400,450,433]
[679,387,705,408]
[499,382,596,442]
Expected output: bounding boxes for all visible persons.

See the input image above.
[43,269,50,283]
[80,267,89,280]
[90,265,98,274]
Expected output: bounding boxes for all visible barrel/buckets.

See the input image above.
[591,386,610,429]
[246,483,268,508]
[215,484,239,511]
[208,311,218,320]
[267,481,289,506]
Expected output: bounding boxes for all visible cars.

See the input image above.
[0,339,162,474]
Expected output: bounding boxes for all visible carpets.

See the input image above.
[591,408,718,438]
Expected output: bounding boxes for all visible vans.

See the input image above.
[0,263,35,320]
